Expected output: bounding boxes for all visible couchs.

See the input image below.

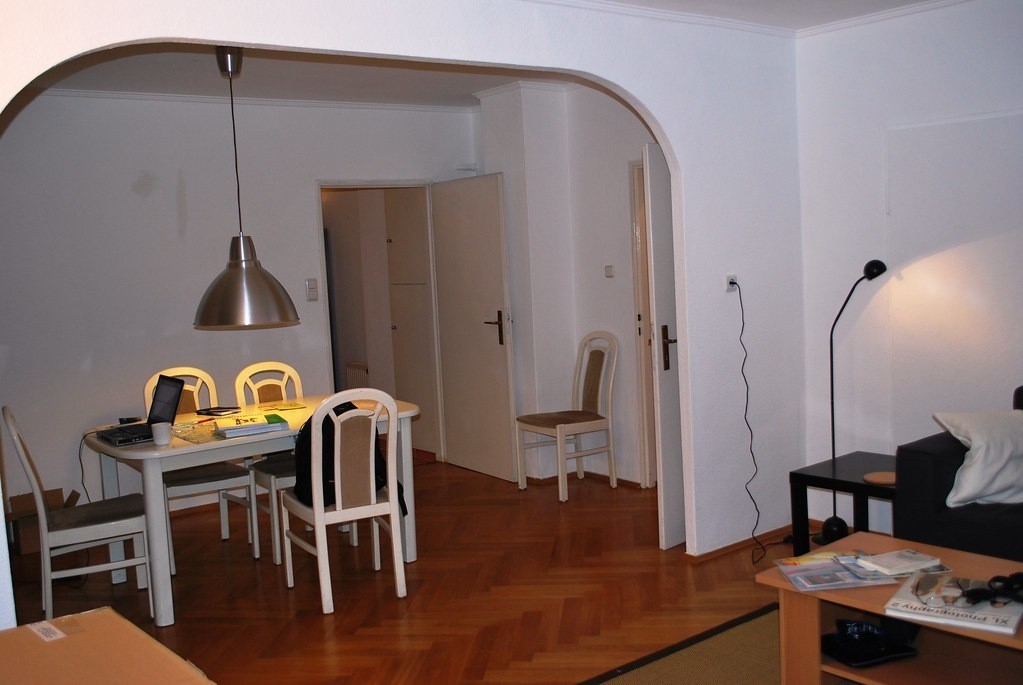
[893,385,1023,559]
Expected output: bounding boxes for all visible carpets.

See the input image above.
[576,599,782,685]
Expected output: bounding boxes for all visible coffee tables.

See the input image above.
[756,532,1023,685]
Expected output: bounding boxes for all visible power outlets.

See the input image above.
[726,274,738,291]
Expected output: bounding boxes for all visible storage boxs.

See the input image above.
[0,606,213,685]
[6,484,82,556]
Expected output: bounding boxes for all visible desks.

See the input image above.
[790,450,896,553]
[86,392,421,625]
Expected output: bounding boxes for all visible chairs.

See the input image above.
[3,405,156,623]
[144,367,260,574]
[515,332,619,502]
[278,386,408,616]
[238,362,359,564]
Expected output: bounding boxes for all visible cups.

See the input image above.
[151,422,175,445]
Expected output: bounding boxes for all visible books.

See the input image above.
[883,571,1023,635]
[195,407,240,415]
[857,546,940,575]
[215,412,290,439]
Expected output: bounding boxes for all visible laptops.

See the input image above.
[96,374,185,447]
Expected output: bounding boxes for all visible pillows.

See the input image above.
[933,408,1023,508]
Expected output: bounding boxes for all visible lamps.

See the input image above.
[817,259,886,542]
[195,45,300,330]
